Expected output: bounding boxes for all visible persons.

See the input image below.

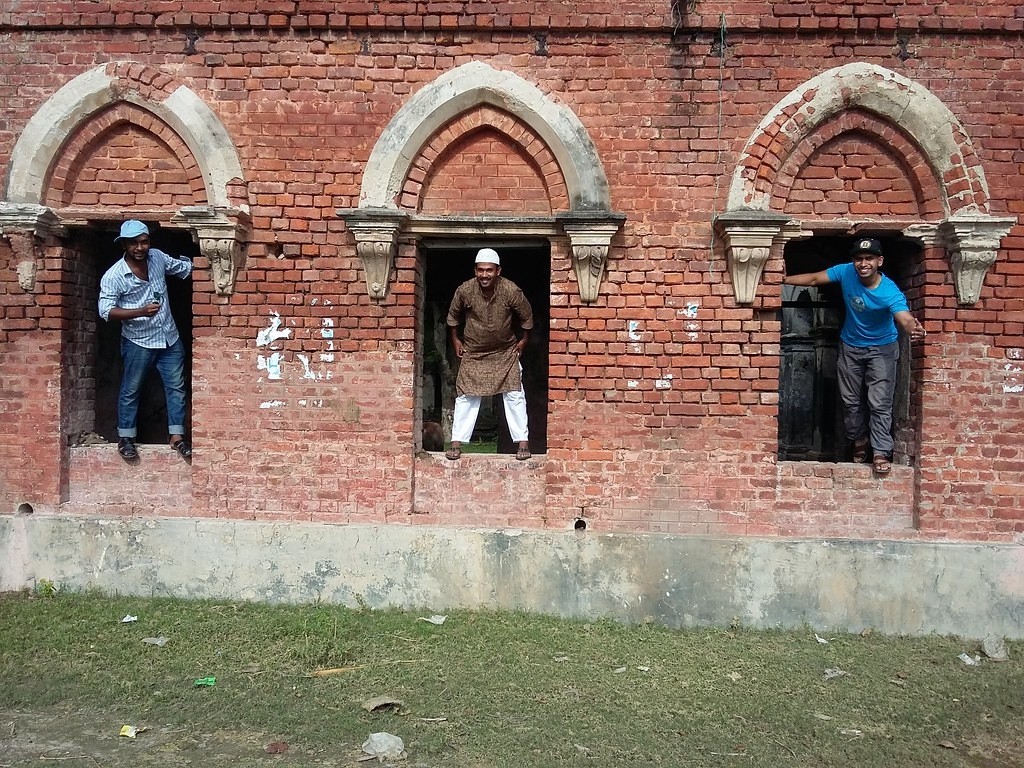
[444,248,535,461]
[781,237,927,474]
[96,219,190,460]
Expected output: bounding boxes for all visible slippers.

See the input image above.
[445,447,461,459]
[517,448,531,459]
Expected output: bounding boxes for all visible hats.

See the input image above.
[113,220,149,242]
[856,238,882,255]
[475,248,499,264]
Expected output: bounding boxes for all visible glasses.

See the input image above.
[152,292,160,304]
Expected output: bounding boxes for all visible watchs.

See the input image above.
[923,327,927,339]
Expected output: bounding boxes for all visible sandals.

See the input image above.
[171,439,191,456]
[118,437,137,458]
[873,456,891,472]
[853,441,867,462]
